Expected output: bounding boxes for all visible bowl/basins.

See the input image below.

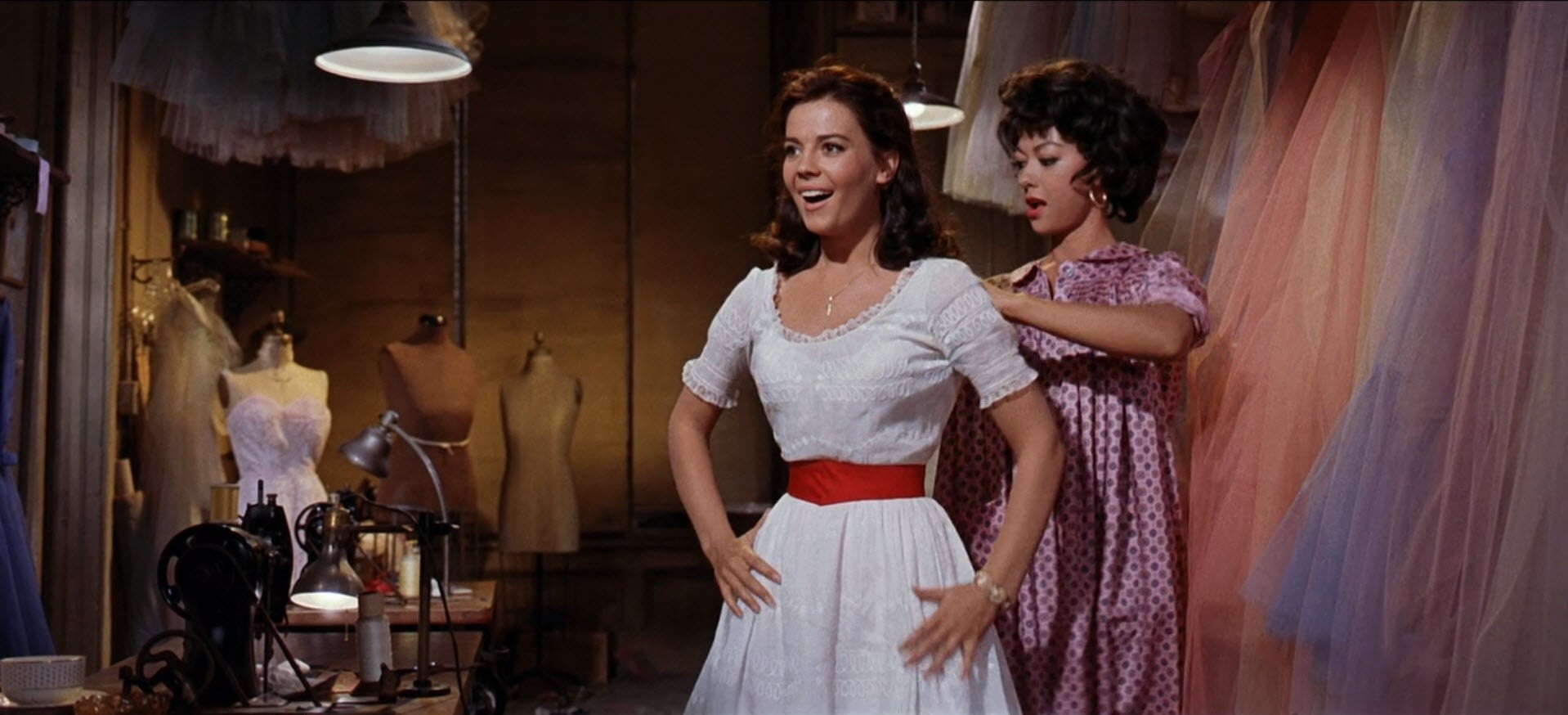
[0,655,86,705]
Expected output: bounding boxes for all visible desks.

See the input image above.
[284,579,497,626]
[54,630,485,715]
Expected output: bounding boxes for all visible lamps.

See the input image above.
[341,411,453,599]
[284,495,450,698]
[891,0,965,129]
[313,0,474,83]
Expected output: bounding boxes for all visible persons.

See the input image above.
[499,331,583,551]
[220,309,332,591]
[936,57,1210,715]
[381,311,478,511]
[668,62,1064,715]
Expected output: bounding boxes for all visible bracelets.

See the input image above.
[976,570,1013,611]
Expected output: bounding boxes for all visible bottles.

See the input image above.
[399,540,420,598]
[354,591,392,684]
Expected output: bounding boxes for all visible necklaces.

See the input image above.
[821,264,875,316]
